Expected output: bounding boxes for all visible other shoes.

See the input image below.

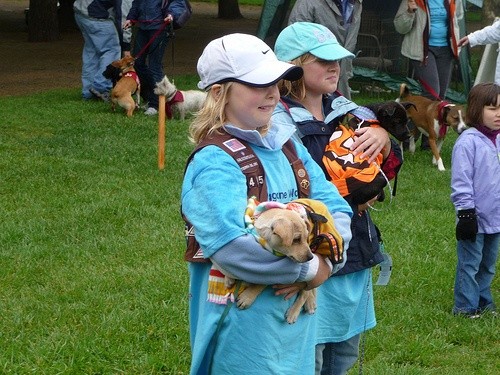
[453,312,480,319]
[140,101,158,116]
[83,85,113,103]
[477,309,500,318]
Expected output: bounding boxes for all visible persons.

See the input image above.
[273,23,403,375]
[395,0,460,149]
[455,6,500,86]
[451,83,500,320]
[72,0,123,101]
[179,32,352,375]
[289,0,365,103]
[123,1,196,116]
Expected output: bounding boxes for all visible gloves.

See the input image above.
[456,208,479,244]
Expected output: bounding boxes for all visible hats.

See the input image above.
[197,33,304,90]
[274,20,356,63]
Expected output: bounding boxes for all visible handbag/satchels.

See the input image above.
[163,0,192,29]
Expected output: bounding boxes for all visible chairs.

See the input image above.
[353,33,392,99]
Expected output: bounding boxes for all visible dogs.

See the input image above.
[395,81,469,173]
[152,72,209,122]
[222,197,333,326]
[320,96,420,202]
[111,56,141,119]
[98,60,122,88]
